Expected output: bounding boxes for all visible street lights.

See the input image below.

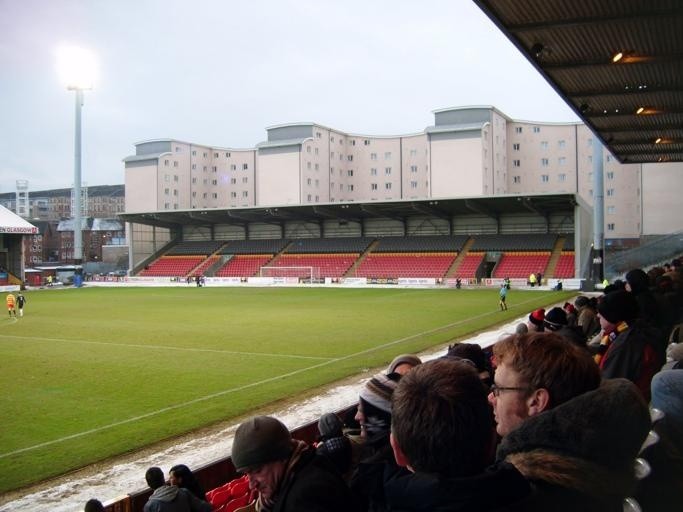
[52,42,101,268]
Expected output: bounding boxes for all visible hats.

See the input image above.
[544,308,568,328]
[448,342,485,370]
[529,307,545,324]
[598,292,633,323]
[626,268,650,291]
[230,417,295,470]
[385,354,423,374]
[318,413,344,437]
[357,373,401,419]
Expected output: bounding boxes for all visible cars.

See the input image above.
[108,269,127,276]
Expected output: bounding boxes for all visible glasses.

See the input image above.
[489,384,533,397]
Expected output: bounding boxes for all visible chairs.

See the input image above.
[623,496,643,512]
[650,408,666,434]
[630,458,652,499]
[205,472,259,512]
[636,430,661,458]
[667,324,682,345]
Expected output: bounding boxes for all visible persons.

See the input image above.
[85,259,683,512]
[6,273,53,318]
